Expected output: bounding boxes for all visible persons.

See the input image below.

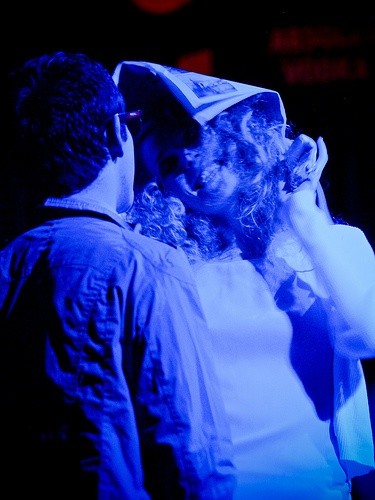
[127,93,375,500]
[0,49,240,499]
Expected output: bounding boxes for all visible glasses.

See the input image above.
[117,107,144,142]
[155,111,229,179]
